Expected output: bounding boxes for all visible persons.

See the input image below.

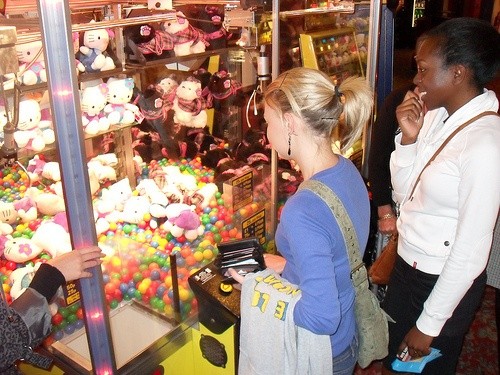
[380,17,500,375]
[0,245,106,375]
[228,67,375,375]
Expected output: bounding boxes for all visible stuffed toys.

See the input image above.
[191,6,241,50]
[194,69,212,101]
[321,34,367,66]
[0,28,141,150]
[133,71,231,158]
[21,155,146,180]
[0,168,218,263]
[158,73,178,109]
[124,9,206,63]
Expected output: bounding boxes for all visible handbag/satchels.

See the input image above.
[295,180,396,369]
[368,231,399,284]
